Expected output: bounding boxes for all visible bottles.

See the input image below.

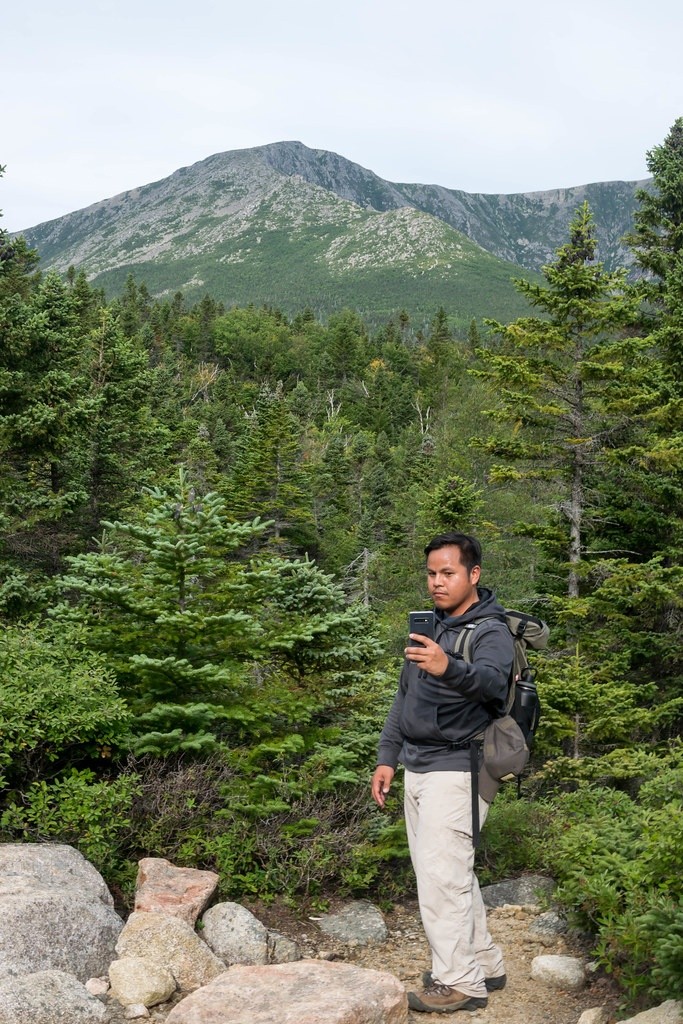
[516,666,539,708]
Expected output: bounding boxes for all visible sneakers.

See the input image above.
[423,970,507,992]
[406,978,487,1013]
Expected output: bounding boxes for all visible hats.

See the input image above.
[476,716,527,801]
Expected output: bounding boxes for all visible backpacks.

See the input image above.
[454,608,550,746]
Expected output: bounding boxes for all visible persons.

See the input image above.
[371,532,518,1013]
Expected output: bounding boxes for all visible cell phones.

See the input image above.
[409,612,435,664]
